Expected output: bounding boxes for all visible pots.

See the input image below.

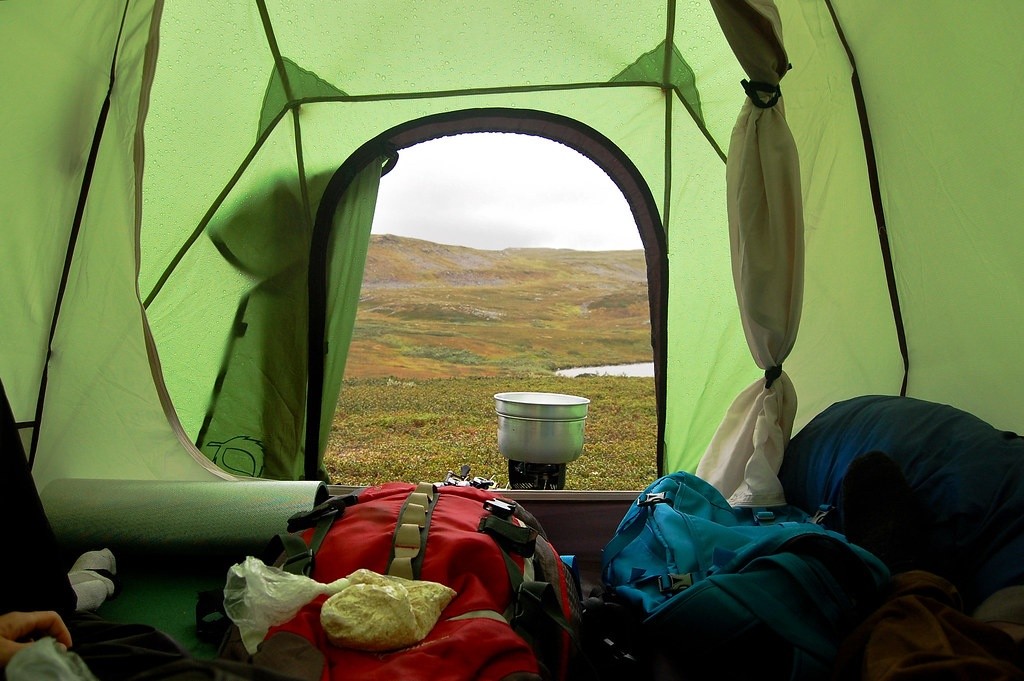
[493,392,591,464]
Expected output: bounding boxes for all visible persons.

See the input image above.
[0,379,217,681]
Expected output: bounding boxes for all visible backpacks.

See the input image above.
[595,470,888,681]
[284,467,578,681]
